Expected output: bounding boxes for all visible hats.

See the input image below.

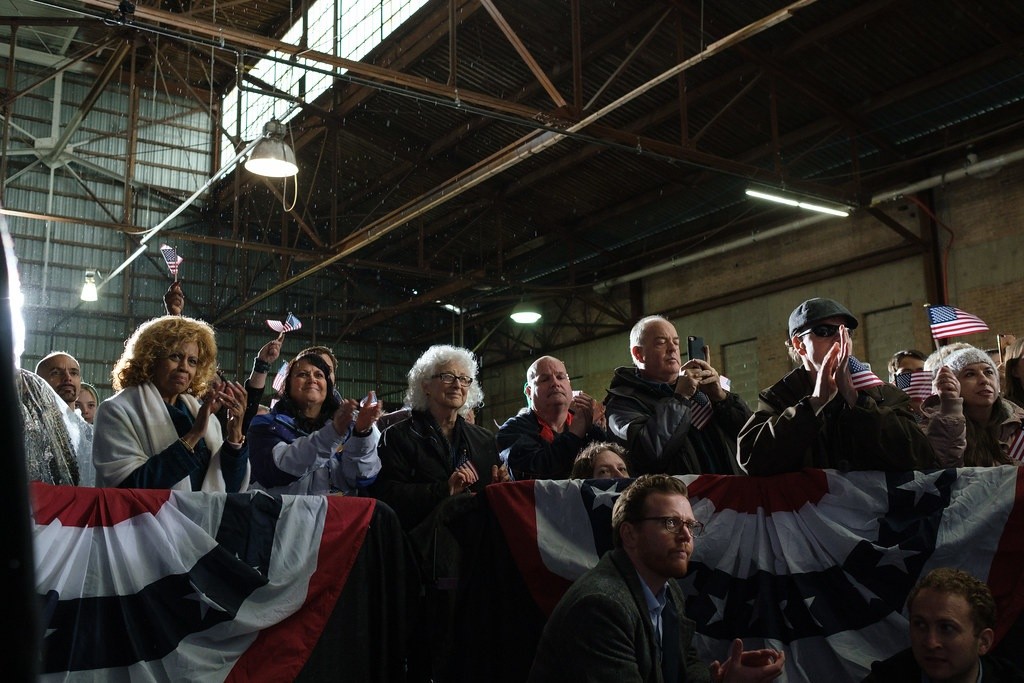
[788,297,859,338]
[924,342,997,378]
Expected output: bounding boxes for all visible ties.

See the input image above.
[658,615,663,661]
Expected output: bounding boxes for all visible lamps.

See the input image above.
[244,119,299,178]
[508,295,542,324]
[80,268,98,301]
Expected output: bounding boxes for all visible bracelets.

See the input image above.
[352,424,373,438]
[253,357,272,374]
[181,437,193,450]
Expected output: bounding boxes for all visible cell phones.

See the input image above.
[687,335,705,369]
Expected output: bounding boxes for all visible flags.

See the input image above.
[160,243,183,275]
[848,355,885,391]
[925,305,990,339]
[894,371,933,398]
[264,313,302,332]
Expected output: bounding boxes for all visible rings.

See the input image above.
[232,402,240,409]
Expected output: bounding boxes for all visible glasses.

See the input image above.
[643,516,704,538]
[432,371,473,387]
[797,324,853,338]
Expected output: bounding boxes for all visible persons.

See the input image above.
[859,568,1024,683]
[93,279,514,496]
[15,349,100,487]
[495,314,755,482]
[887,336,1024,469]
[530,473,786,683]
[735,296,936,476]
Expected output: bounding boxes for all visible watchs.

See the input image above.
[228,435,245,444]
